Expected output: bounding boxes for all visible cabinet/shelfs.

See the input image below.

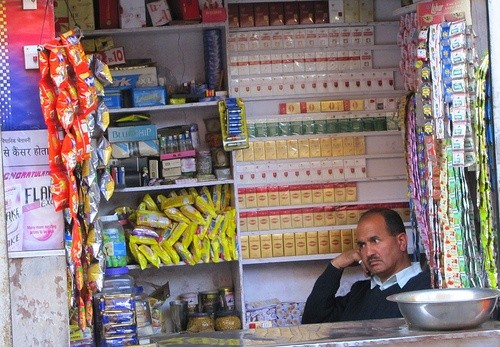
[55,0,443,330]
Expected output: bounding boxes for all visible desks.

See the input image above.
[151,318,499,347]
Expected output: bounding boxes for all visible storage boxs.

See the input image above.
[55,0,231,159]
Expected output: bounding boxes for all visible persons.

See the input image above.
[299,207,432,325]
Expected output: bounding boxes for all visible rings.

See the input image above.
[357,259,364,265]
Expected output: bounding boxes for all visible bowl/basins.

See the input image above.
[386,288,500,330]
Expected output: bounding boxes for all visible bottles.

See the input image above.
[197,151,212,175]
